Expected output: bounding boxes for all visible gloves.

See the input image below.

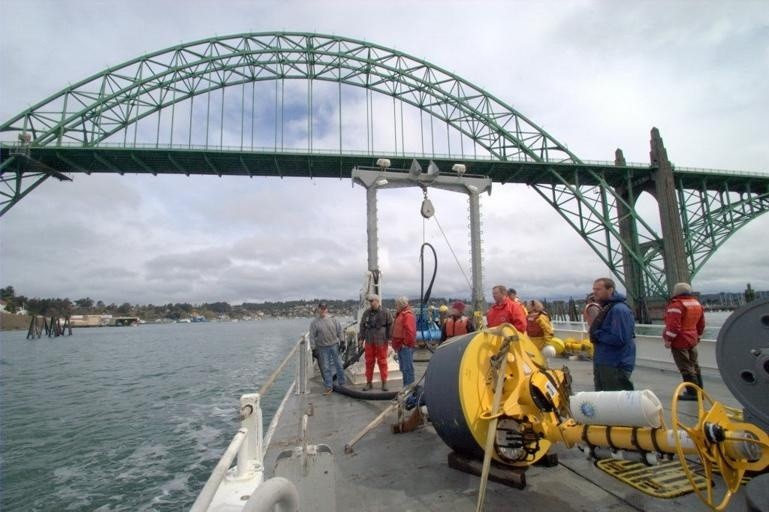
[313,349,319,359]
[339,341,345,352]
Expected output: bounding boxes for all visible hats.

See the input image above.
[319,301,327,309]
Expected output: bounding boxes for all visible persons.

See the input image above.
[428,286,554,368]
[589,278,636,391]
[662,282,705,401]
[357,294,393,391]
[309,301,345,395]
[586,292,602,325]
[390,296,417,411]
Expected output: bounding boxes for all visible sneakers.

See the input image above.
[382,384,389,391]
[678,394,697,400]
[323,387,333,395]
[363,383,372,391]
